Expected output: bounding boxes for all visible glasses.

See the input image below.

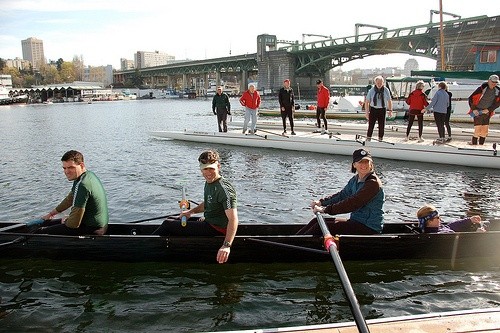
[433,217,440,219]
[200,158,220,164]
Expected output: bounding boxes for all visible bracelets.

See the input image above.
[50,212,53,216]
[389,109,392,112]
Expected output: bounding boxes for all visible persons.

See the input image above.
[443,84,452,142]
[364,76,393,142]
[416,206,486,234]
[211,86,231,132]
[405,81,429,142]
[30,149,109,235]
[151,150,239,263]
[239,84,261,135]
[295,149,385,235]
[278,79,296,135]
[467,75,500,145]
[420,82,449,139]
[315,79,330,132]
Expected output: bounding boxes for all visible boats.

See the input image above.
[0,220,500,264]
[151,76,499,169]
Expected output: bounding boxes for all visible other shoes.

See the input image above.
[420,138,424,142]
[324,130,328,134]
[291,132,296,135]
[446,138,452,142]
[313,130,321,133]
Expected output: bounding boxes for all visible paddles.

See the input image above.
[0,219,44,231]
[361,135,396,146]
[315,211,371,333]
[320,122,342,127]
[357,140,366,146]
[254,134,267,140]
[181,187,186,227]
[258,129,289,138]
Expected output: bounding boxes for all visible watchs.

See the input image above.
[223,241,231,247]
[63,218,68,222]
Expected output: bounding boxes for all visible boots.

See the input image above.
[472,137,478,145]
[479,137,485,145]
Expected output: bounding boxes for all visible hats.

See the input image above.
[489,75,499,83]
[198,151,219,169]
[316,79,322,84]
[217,86,222,89]
[353,149,373,163]
